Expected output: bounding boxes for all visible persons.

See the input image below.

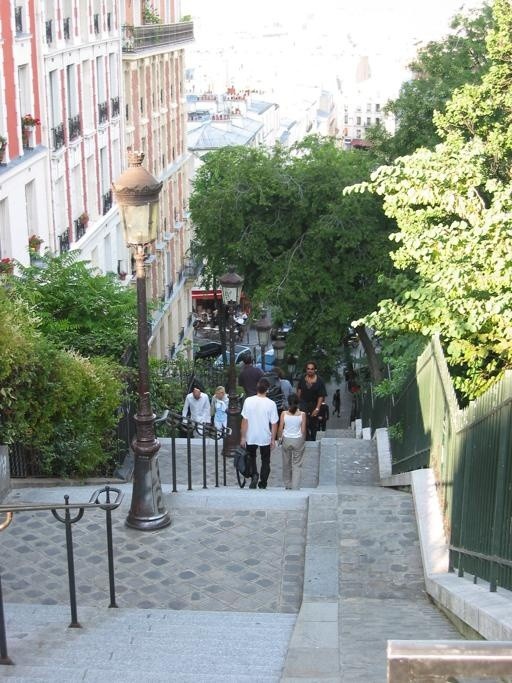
[260,372,286,440]
[317,395,330,431]
[331,388,341,417]
[296,359,328,440]
[210,384,229,441]
[180,383,211,440]
[239,377,281,489]
[237,353,265,397]
[278,391,308,491]
[270,367,295,409]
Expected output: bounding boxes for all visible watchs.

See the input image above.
[315,407,319,411]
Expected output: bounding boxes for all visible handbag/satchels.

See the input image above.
[234,442,253,488]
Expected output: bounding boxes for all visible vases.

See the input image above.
[23,129,32,144]
[32,243,40,252]
[5,268,13,274]
[0,151,5,163]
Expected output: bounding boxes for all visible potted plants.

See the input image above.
[119,271,127,280]
[80,214,90,224]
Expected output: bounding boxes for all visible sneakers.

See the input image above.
[249,472,259,488]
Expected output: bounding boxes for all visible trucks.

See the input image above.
[249,349,274,374]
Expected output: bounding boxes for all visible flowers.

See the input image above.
[21,114,41,129]
[0,136,8,150]
[0,258,14,271]
[29,235,44,245]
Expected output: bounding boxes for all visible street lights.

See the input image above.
[285,352,298,390]
[271,339,288,384]
[217,264,250,458]
[109,143,178,534]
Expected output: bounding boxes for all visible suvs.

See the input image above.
[213,345,251,372]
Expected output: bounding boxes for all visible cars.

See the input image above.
[313,331,359,368]
[192,341,227,363]
[268,319,296,340]
